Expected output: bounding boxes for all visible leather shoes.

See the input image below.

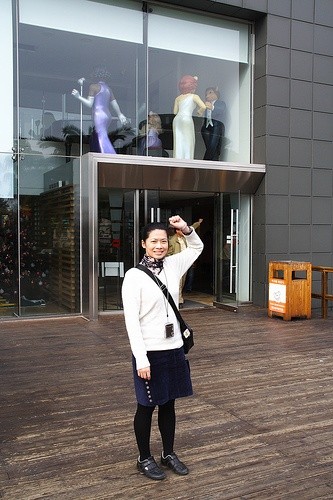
[137,456,167,480]
[161,451,189,475]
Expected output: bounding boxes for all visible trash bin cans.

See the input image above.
[269,261,312,322]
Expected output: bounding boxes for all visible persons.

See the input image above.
[138,114,163,157]
[166,218,204,309]
[71,68,126,154]
[200,86,226,161]
[172,74,206,160]
[121,215,205,480]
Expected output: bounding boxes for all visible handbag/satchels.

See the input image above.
[180,321,195,354]
